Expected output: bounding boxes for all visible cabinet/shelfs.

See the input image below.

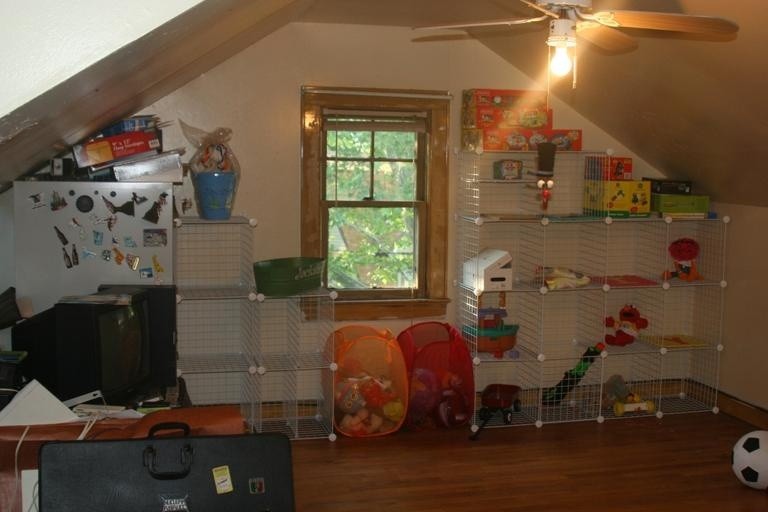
[173,216,339,443]
[454,147,730,431]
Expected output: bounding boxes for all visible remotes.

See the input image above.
[142,402,169,407]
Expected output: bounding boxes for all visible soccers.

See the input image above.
[731,431,768,489]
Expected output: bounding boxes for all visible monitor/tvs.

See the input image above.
[11,287,153,405]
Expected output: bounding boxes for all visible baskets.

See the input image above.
[642,176,710,213]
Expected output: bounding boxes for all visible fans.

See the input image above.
[411,0,739,50]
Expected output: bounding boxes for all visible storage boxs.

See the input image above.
[586,156,632,181]
[583,179,651,218]
[461,88,583,151]
[399,321,474,435]
[321,324,408,439]
[653,194,712,212]
[463,247,515,292]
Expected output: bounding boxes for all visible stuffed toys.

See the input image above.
[604,304,648,345]
[542,342,605,406]
[662,238,704,281]
[334,374,404,435]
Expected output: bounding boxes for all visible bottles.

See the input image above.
[53,225,80,269]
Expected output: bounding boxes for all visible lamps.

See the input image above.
[546,19,577,77]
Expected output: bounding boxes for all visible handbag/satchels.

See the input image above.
[38,420,294,512]
